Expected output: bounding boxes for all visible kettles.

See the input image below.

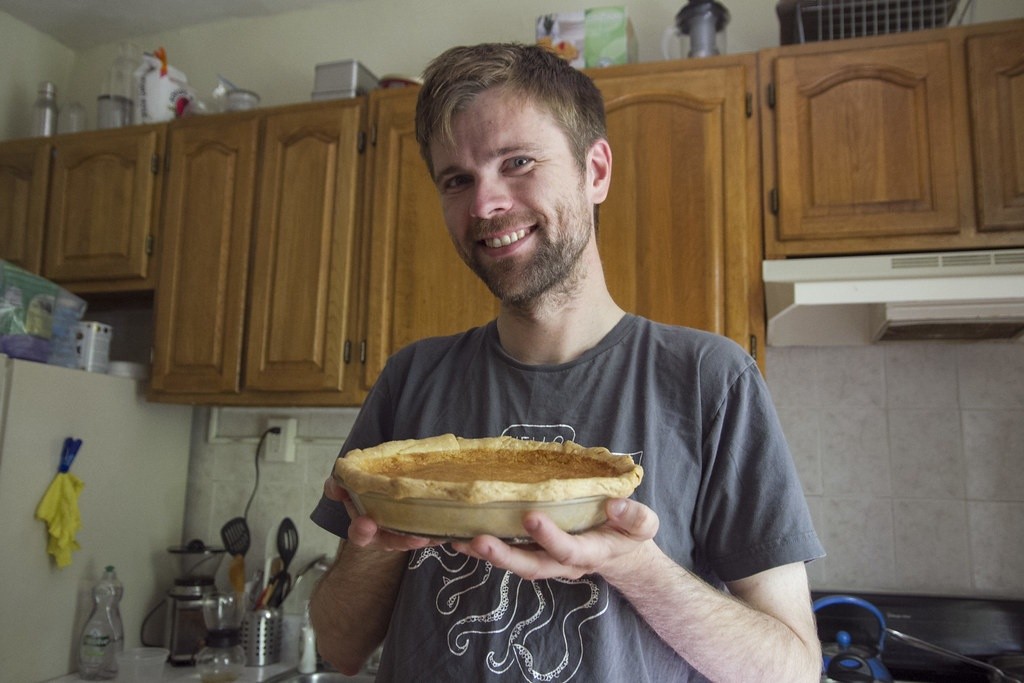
[797,593,895,683]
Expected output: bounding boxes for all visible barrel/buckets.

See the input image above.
[70,321,112,376]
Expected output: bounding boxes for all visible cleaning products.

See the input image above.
[78,565,125,682]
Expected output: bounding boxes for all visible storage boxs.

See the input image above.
[310,59,380,99]
[535,5,638,69]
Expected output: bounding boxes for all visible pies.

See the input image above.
[335,432,644,540]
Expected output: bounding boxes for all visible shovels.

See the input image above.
[277,518,299,602]
[228,553,245,589]
[221,517,251,559]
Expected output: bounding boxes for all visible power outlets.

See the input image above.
[265,418,297,462]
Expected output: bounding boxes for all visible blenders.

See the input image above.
[161,540,226,669]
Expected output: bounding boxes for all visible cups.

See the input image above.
[116,647,169,683]
[222,88,262,110]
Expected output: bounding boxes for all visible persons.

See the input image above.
[307,44,823,683]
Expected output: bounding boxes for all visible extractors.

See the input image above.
[760,248,1024,349]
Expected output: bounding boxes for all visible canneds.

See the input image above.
[75,320,113,374]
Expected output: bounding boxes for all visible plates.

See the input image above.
[337,484,628,546]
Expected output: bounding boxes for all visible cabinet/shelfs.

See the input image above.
[0,121,168,310]
[355,51,764,405]
[758,19,1024,259]
[144,98,366,408]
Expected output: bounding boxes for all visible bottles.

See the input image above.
[296,596,320,672]
[77,566,125,681]
[94,94,132,128]
[27,81,59,140]
